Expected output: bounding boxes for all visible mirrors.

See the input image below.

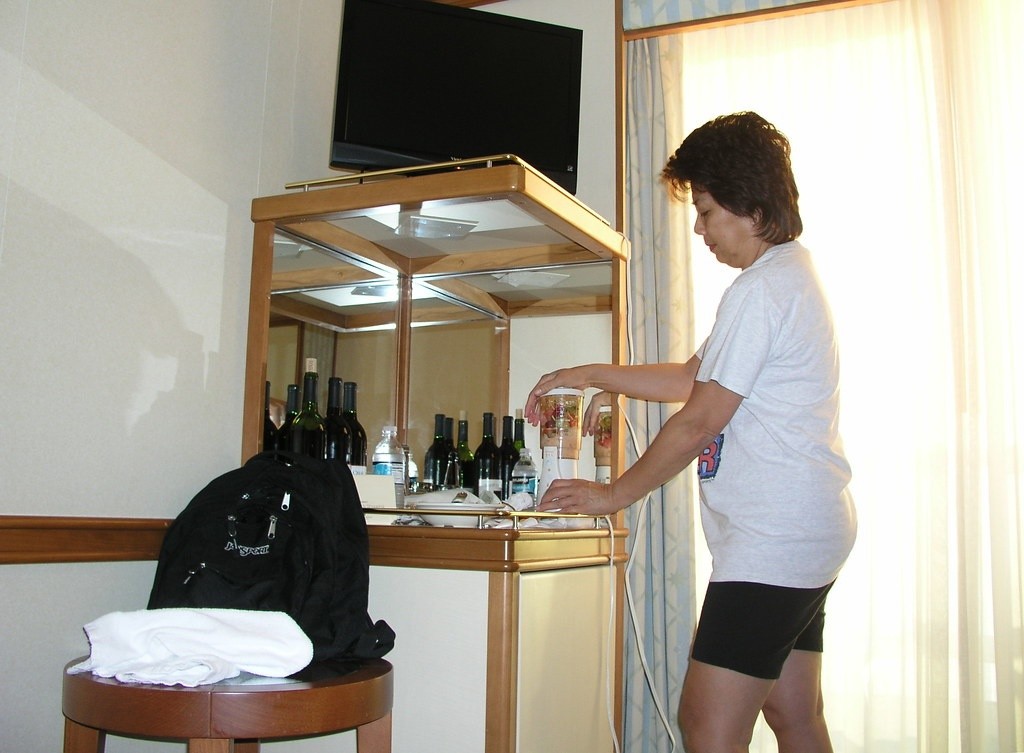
[265,225,404,475]
[407,258,611,505]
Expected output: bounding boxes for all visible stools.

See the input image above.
[62,654,394,753]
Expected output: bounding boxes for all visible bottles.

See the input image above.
[261,374,368,473]
[371,425,405,510]
[536,446,562,514]
[423,411,525,503]
[403,446,420,494]
[510,451,538,513]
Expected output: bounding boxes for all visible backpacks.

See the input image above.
[147,448,395,678]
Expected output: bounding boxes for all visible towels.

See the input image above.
[62,608,314,689]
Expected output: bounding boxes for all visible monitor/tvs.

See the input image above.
[329,0,583,194]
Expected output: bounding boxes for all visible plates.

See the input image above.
[416,503,505,528]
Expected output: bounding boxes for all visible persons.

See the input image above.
[581,389,613,437]
[523,111,856,753]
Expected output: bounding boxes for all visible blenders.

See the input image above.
[537,387,584,515]
[592,407,613,488]
